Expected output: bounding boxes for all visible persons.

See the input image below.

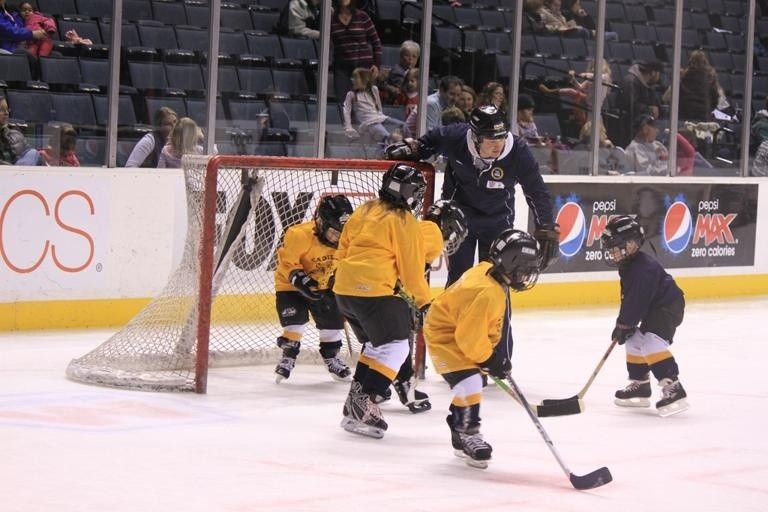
[602,215,690,418]
[751,139,768,176]
[432,0,473,8]
[370,199,469,414]
[624,114,679,176]
[377,40,433,105]
[403,76,463,142]
[274,195,355,384]
[664,127,696,176]
[608,56,667,150]
[754,31,766,71]
[19,2,57,90]
[343,68,413,147]
[40,126,80,167]
[662,50,740,160]
[564,121,648,175]
[0,0,47,82]
[126,107,178,168]
[0,130,47,166]
[540,57,613,121]
[749,97,768,157]
[455,85,476,116]
[0,94,16,165]
[331,163,435,439]
[566,0,618,41]
[522,0,545,36]
[423,229,543,470]
[441,107,465,125]
[393,68,420,119]
[330,0,383,126]
[383,105,560,388]
[156,118,218,168]
[289,0,334,39]
[517,93,550,147]
[538,0,575,33]
[477,82,506,108]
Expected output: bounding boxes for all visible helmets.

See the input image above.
[468,105,510,161]
[600,216,645,268]
[422,198,469,257]
[379,164,428,216]
[488,228,544,293]
[311,194,355,249]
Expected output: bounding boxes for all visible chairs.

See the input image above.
[1,1,387,167]
[380,0,768,175]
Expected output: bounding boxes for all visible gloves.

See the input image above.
[484,354,514,380]
[288,268,324,301]
[610,323,637,346]
[533,222,562,271]
[384,137,424,163]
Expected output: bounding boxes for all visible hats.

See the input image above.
[645,61,664,77]
[632,114,661,134]
[518,93,535,110]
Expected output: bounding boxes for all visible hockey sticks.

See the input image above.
[397,290,581,417]
[542,336,619,405]
[508,372,612,491]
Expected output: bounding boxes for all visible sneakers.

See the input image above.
[275,348,300,379]
[450,422,492,461]
[615,379,652,399]
[394,378,429,406]
[656,380,686,408]
[342,384,389,431]
[323,356,352,377]
